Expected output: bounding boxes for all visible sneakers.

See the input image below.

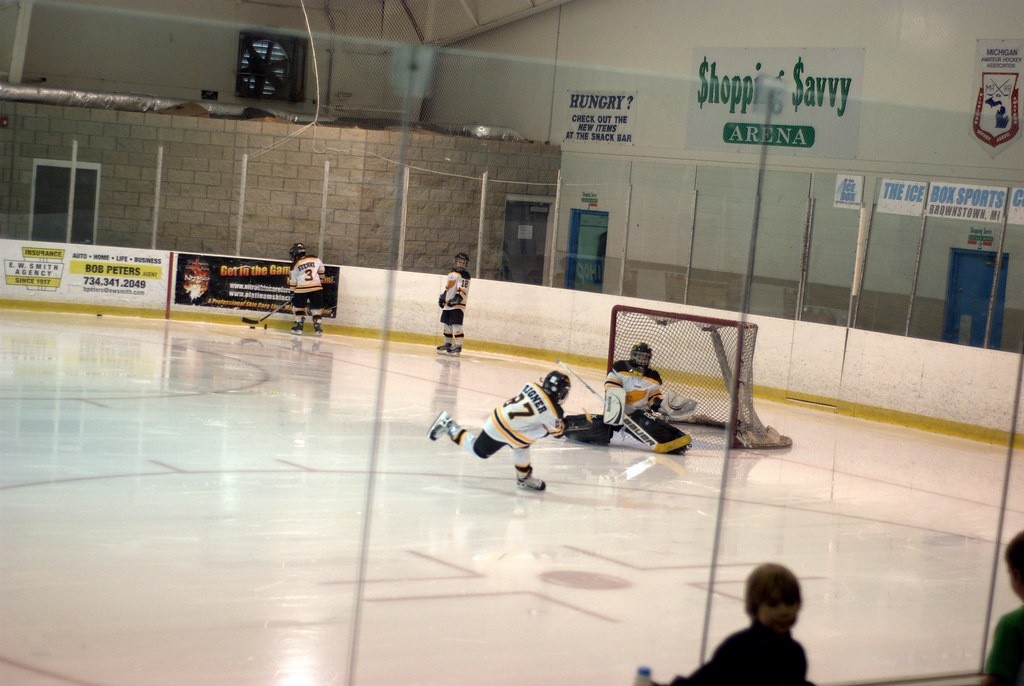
[314,322,323,336]
[291,322,303,335]
[446,344,462,356]
[436,343,452,354]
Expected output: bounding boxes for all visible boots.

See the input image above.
[429,410,465,444]
[515,467,545,490]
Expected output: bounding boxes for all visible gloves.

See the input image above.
[439,298,445,308]
[447,300,453,307]
[562,419,569,431]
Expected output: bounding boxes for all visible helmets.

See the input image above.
[289,243,306,259]
[454,253,469,271]
[630,342,652,367]
[543,371,571,400]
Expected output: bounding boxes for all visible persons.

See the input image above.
[435,252,471,357]
[653,563,815,686]
[564,342,692,456]
[976,529,1024,686]
[427,370,571,491]
[286,243,326,337]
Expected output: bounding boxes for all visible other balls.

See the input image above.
[97,314,102,316]
[265,324,267,329]
[250,327,255,328]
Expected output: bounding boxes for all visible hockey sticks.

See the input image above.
[239,299,292,324]
[566,407,594,432]
[555,357,693,454]
